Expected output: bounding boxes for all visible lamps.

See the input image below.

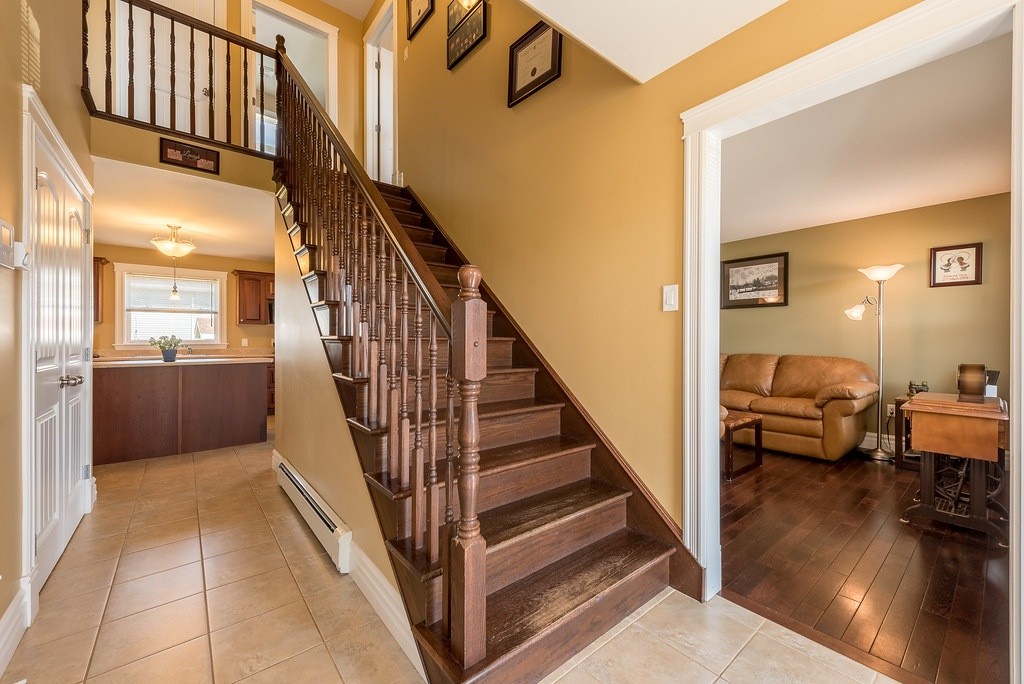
[844,263,905,460]
[147,224,197,302]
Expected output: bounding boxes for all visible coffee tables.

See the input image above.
[720,411,764,481]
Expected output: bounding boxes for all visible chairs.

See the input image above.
[923,370,1000,504]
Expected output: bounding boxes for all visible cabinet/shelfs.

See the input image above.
[94,257,110,324]
[232,269,276,326]
[266,356,275,413]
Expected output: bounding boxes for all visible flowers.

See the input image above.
[149,336,189,351]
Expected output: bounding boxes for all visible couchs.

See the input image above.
[719,352,879,460]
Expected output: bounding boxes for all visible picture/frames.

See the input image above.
[507,20,562,109]
[447,0,482,36]
[447,1,487,70]
[720,252,789,309]
[406,0,434,40]
[930,243,984,288]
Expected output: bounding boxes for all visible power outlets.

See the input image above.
[271,339,275,346]
[886,404,895,417]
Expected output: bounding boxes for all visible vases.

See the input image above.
[162,350,177,362]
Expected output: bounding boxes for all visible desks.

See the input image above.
[894,394,922,472]
